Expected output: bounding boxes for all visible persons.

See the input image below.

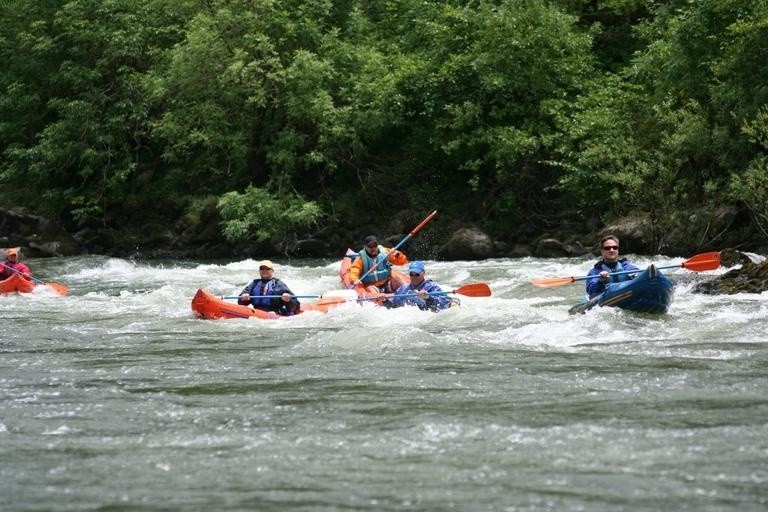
[0,248,32,281]
[586,235,640,300]
[347,235,407,310]
[377,261,451,313]
[238,260,296,312]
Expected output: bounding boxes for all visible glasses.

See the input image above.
[603,245,619,252]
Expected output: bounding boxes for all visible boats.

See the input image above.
[337,246,411,306]
[0,272,68,298]
[581,264,674,317]
[189,287,348,322]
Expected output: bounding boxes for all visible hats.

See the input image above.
[364,235,378,246]
[408,261,425,275]
[258,259,275,271]
[6,248,18,257]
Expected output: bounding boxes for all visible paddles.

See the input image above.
[531,251,721,289]
[0,263,67,296]
[215,288,358,301]
[315,282,491,312]
